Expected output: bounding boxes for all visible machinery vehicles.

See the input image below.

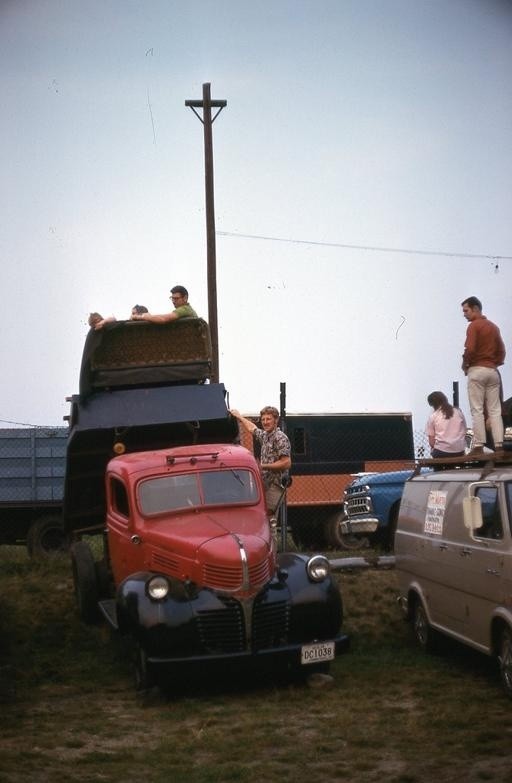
[58,310,347,700]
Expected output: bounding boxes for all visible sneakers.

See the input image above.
[496,446,504,452]
[467,445,483,455]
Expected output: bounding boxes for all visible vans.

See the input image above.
[395,450,511,695]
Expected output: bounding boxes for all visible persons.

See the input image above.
[460,296,512,455]
[143,285,197,325]
[425,391,467,472]
[229,406,291,541]
[88,313,116,331]
[132,305,148,321]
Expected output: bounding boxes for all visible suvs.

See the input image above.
[340,429,511,548]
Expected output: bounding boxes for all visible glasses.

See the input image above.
[169,296,184,301]
